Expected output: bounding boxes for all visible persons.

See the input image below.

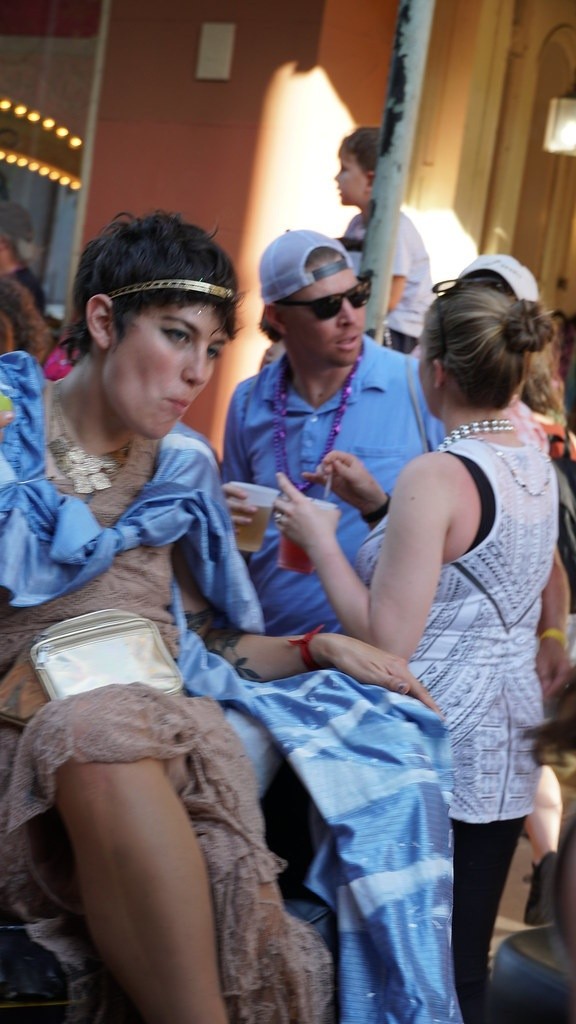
[0,125,575,1024]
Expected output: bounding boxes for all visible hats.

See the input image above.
[0,199,38,262]
[455,254,541,305]
[260,230,353,304]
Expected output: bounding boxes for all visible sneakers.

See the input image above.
[524,852,556,928]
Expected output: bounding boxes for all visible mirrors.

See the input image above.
[0,148,79,338]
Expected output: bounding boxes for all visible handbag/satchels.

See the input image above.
[27,605,184,707]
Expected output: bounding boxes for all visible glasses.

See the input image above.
[274,268,375,320]
[432,276,508,360]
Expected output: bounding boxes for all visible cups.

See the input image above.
[279,497,338,574]
[228,482,280,553]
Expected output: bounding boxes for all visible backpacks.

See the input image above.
[550,421,576,615]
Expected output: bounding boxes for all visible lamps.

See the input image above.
[541,83,575,158]
